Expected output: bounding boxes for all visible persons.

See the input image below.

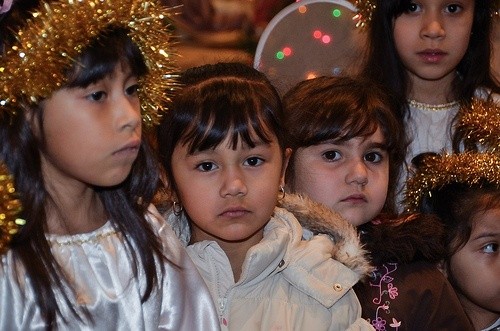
[360,0,500,219]
[280,75,478,331]
[156,61,377,331]
[0,2,224,331]
[421,178,500,331]
